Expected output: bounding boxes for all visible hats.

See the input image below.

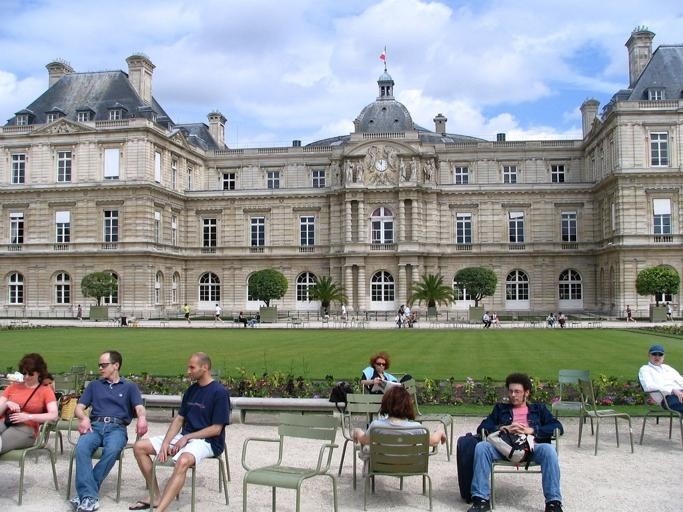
[648,344,664,353]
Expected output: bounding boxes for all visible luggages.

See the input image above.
[455,432,483,504]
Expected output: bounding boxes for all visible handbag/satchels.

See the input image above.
[4,411,24,427]
[330,382,355,404]
[486,424,530,462]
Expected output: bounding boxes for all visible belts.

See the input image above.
[90,416,126,425]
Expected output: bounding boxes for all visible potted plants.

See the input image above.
[247,269,290,323]
[455,268,498,324]
[80,271,118,321]
[403,272,456,317]
[635,265,677,323]
[306,276,346,319]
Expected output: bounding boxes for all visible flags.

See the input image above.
[378,44,387,65]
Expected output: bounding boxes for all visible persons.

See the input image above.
[182,303,190,323]
[557,311,565,328]
[359,350,397,394]
[38,373,62,402]
[625,305,636,323]
[213,304,223,323]
[638,344,682,413]
[323,309,329,318]
[76,304,83,320]
[396,304,416,324]
[238,311,246,327]
[69,350,147,512]
[248,311,260,327]
[546,312,555,327]
[0,353,59,456]
[352,386,446,446]
[128,351,230,512]
[466,372,563,512]
[340,303,345,316]
[491,311,501,328]
[664,302,675,322]
[481,311,490,329]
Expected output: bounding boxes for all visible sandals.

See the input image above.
[128,499,158,510]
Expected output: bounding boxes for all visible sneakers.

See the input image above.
[466,497,489,512]
[544,500,563,512]
[68,495,100,512]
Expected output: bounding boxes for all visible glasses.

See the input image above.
[374,362,386,366]
[651,352,663,356]
[97,362,114,368]
[22,371,35,377]
